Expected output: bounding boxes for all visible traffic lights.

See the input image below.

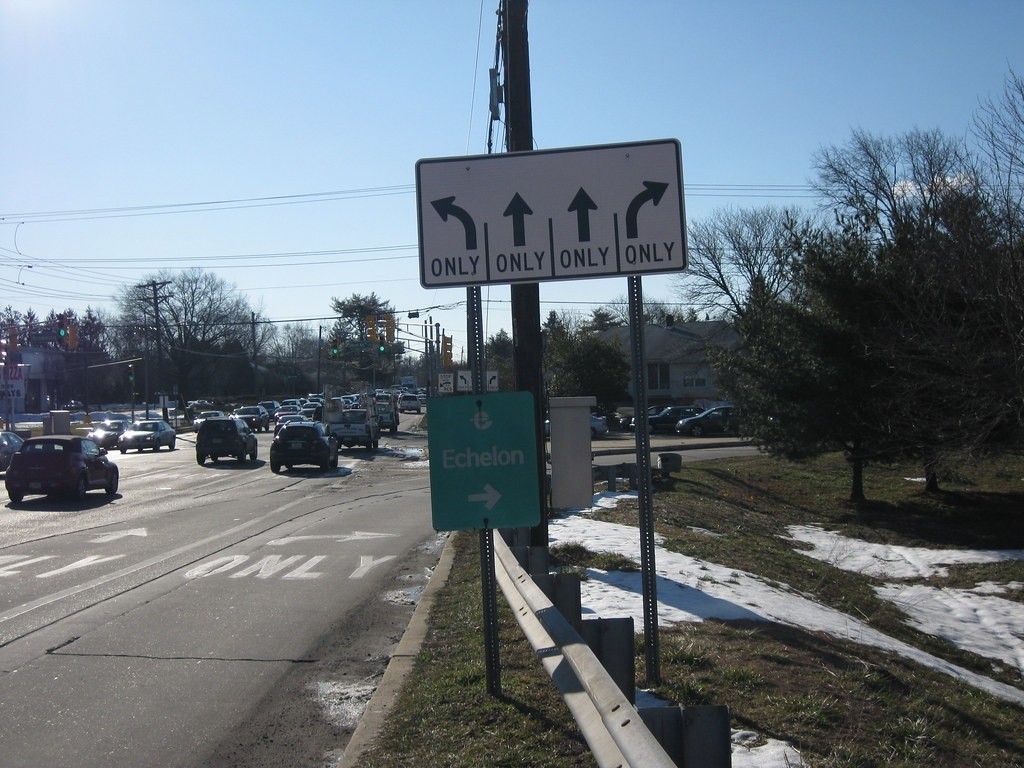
[383,314,395,342]
[57,315,67,337]
[379,335,385,353]
[332,338,338,356]
[443,335,453,368]
[128,364,134,385]
[366,315,378,342]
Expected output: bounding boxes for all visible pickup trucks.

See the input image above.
[375,403,399,433]
[326,409,381,452]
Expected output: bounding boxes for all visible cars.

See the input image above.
[190,376,427,433]
[5,435,118,502]
[621,404,739,437]
[117,420,176,453]
[86,420,131,450]
[0,432,25,472]
[544,415,609,440]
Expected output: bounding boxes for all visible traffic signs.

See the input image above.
[413,138,690,289]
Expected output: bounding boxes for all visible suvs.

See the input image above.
[270,420,338,473]
[196,417,257,465]
